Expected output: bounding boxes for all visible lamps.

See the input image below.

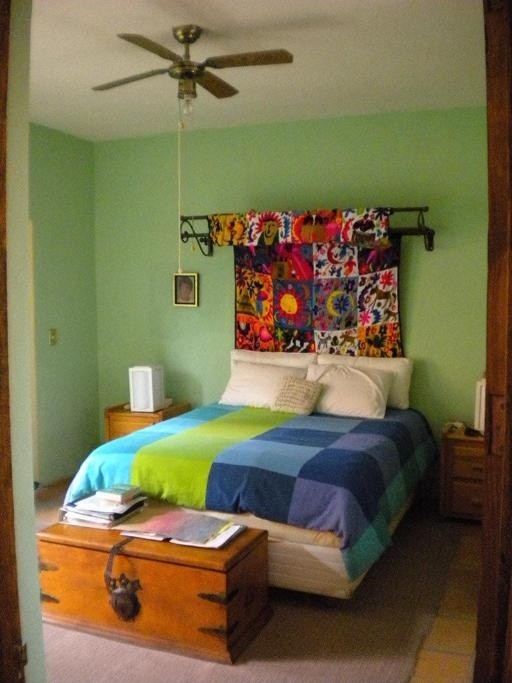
[175,78,198,115]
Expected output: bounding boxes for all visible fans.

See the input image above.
[91,23,296,101]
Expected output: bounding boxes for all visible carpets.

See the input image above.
[36,491,465,681]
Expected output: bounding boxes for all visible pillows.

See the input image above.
[270,375,325,416]
[230,347,317,368]
[317,353,415,410]
[217,359,308,409]
[305,363,395,419]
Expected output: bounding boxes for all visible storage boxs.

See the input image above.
[35,511,275,663]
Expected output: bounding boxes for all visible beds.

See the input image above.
[56,345,439,613]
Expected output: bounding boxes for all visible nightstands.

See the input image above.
[440,420,485,522]
[103,400,189,443]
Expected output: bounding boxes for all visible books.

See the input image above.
[63,480,147,529]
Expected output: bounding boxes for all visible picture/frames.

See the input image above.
[172,271,199,307]
